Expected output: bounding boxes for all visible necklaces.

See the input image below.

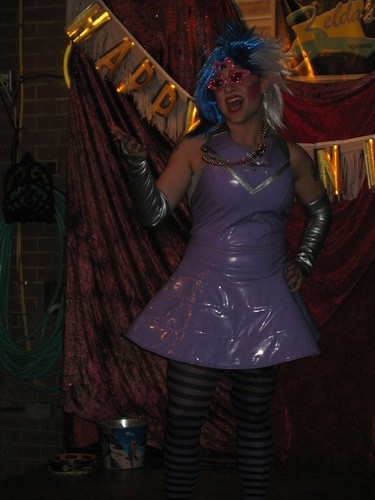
[199,123,268,166]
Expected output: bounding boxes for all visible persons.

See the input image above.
[109,41,332,500]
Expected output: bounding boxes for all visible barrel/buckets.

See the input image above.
[97,418,152,470]
